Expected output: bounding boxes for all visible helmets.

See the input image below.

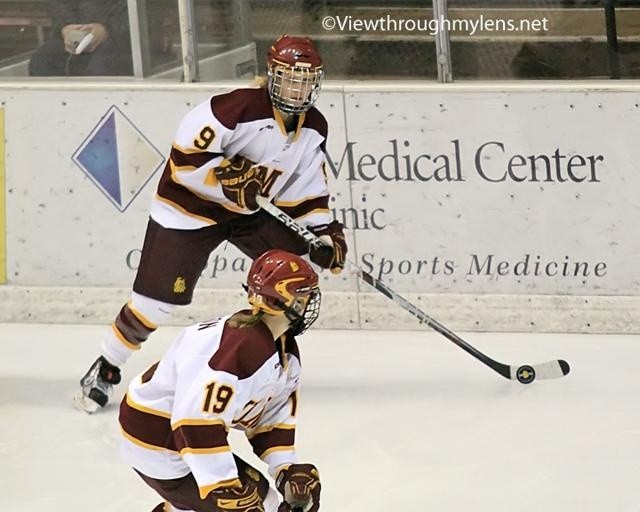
[266,34,324,116]
[247,249,321,345]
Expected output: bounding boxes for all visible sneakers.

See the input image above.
[81,356,121,408]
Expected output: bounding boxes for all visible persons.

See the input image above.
[76,35,346,411]
[117,249,321,508]
[29,1,166,76]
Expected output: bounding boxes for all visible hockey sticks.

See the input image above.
[253,195,570,384]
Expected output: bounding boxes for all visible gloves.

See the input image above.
[276,463,321,512]
[306,220,348,274]
[210,479,265,512]
[214,156,263,210]
